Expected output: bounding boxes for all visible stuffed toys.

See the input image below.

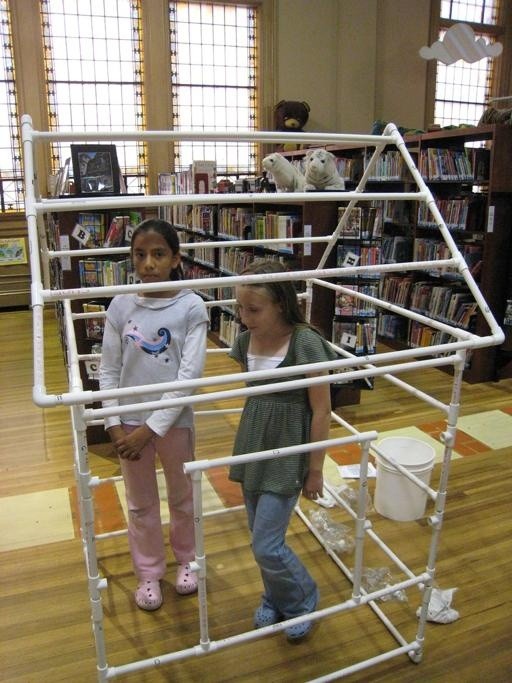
[272,98,312,152]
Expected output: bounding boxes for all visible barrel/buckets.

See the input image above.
[374,438,435,522]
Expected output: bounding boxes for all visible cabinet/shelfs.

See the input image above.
[43,192,151,444]
[263,125,511,385]
[157,173,385,412]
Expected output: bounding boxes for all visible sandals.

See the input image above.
[135,563,198,611]
[254,605,315,639]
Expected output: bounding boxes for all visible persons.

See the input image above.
[98,215,211,613]
[225,259,338,641]
